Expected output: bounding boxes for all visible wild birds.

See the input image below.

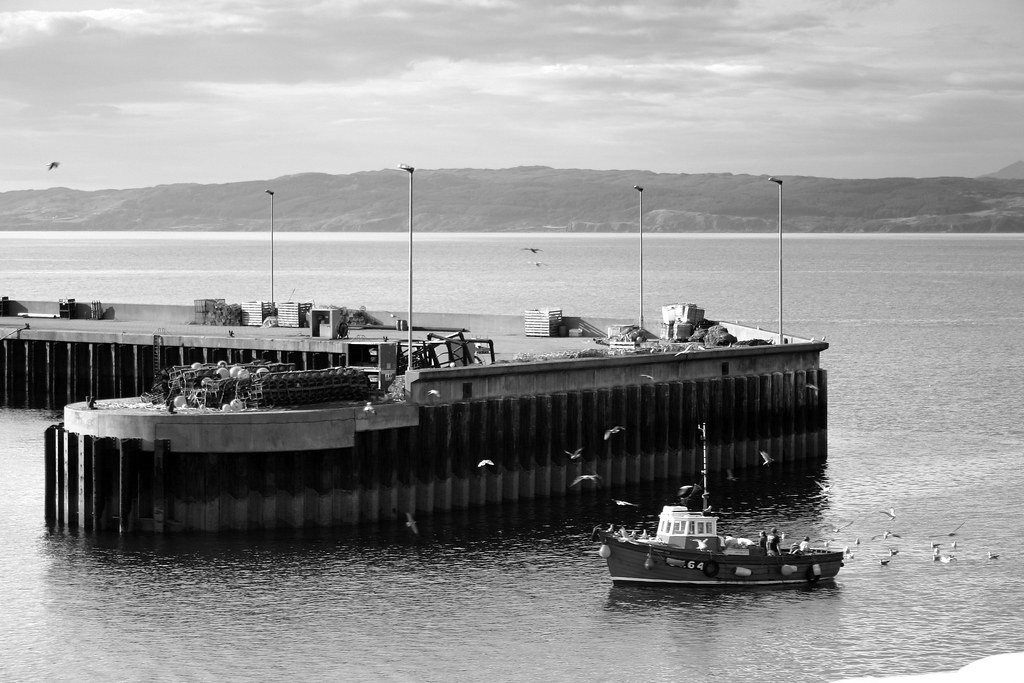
[610,499,639,507]
[604,522,649,540]
[805,382,820,393]
[405,511,419,535]
[640,374,655,381]
[530,260,550,269]
[692,538,708,550]
[674,343,694,357]
[879,507,897,521]
[564,447,583,460]
[929,522,1001,563]
[523,246,544,254]
[812,521,900,565]
[427,389,441,398]
[814,479,835,494]
[46,160,60,170]
[477,459,494,467]
[363,399,378,417]
[602,425,626,440]
[759,449,775,467]
[569,474,604,487]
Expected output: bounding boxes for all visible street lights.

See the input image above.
[634,184,645,330]
[263,189,276,317]
[397,163,415,369]
[767,175,785,345]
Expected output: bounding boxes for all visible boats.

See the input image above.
[594,421,844,589]
